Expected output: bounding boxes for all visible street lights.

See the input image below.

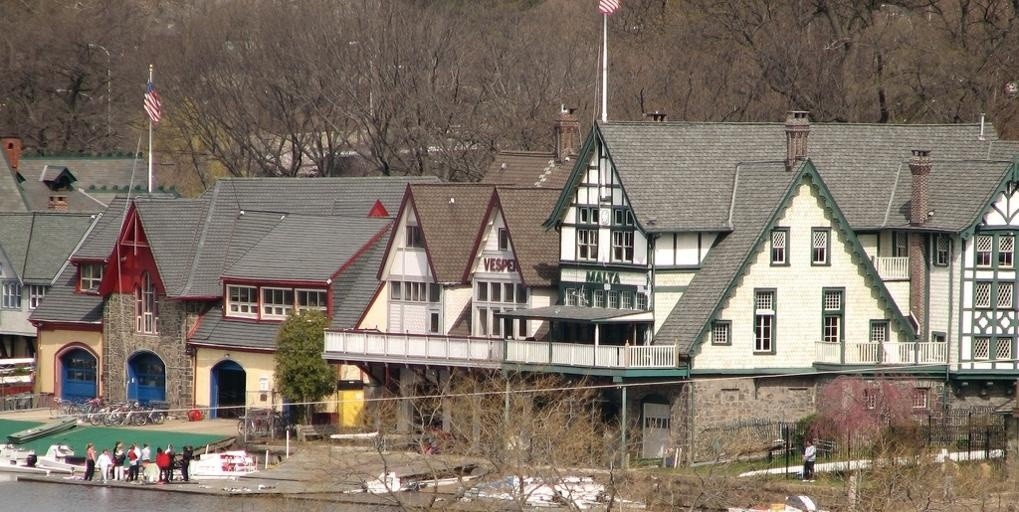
[88,41,113,138]
[347,40,373,118]
[56,87,96,138]
[925,407,936,458]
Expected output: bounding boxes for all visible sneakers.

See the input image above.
[802,480,815,482]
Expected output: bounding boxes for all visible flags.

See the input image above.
[596,0,622,18]
[142,79,164,129]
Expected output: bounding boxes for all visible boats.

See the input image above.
[0,441,262,480]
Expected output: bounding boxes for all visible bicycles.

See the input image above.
[237,408,292,438]
[48,396,167,428]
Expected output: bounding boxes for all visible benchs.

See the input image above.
[296,424,335,441]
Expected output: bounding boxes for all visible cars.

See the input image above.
[0,362,35,376]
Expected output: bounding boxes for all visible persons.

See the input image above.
[800,438,818,482]
[85,441,194,485]
